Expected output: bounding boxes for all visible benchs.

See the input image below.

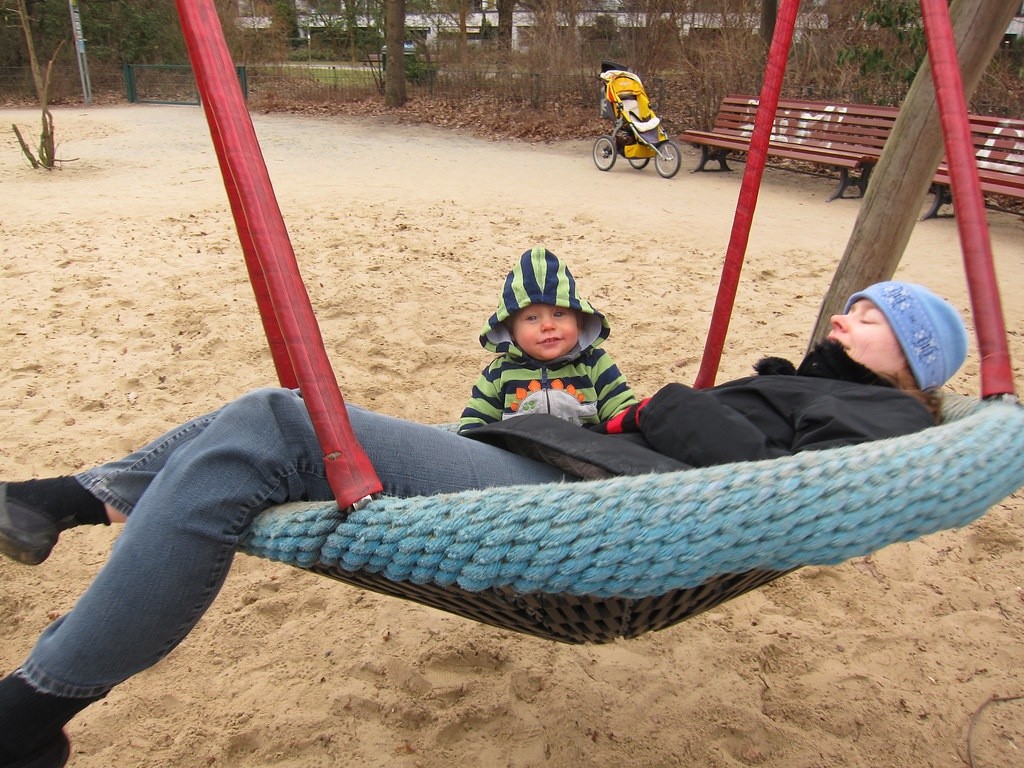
[919,113,1024,222]
[679,94,901,203]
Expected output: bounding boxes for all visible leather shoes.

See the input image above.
[0,481,76,567]
[4,732,70,768]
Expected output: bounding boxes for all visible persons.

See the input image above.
[0,281,969,768]
[458,248,635,436]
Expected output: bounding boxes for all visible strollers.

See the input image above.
[592,70,682,179]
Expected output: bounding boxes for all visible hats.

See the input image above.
[844,281,967,394]
